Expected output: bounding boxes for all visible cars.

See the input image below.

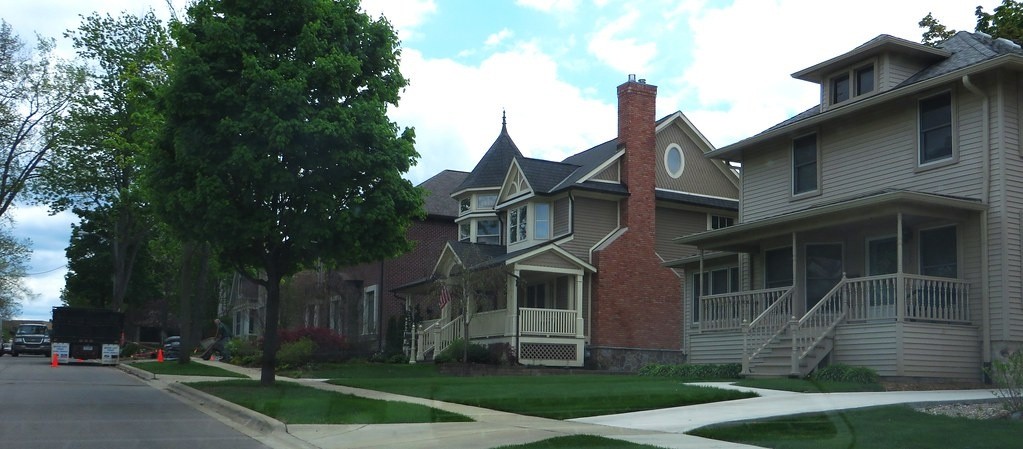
[0,339,18,357]
[163,335,180,348]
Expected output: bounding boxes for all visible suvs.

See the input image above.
[11,324,52,357]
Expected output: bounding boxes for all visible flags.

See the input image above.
[438,285,450,309]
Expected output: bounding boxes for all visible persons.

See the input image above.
[35,327,39,334]
[215,319,231,361]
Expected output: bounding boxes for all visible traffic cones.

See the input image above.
[50,353,60,367]
[157,348,165,362]
